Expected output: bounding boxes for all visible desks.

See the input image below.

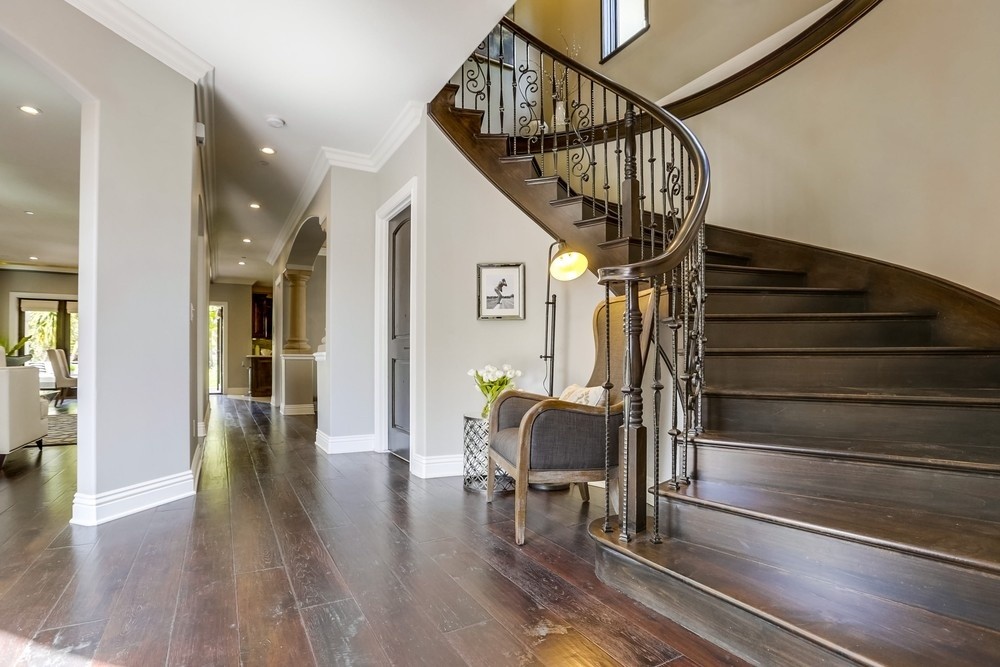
[463,414,516,494]
[39,389,61,404]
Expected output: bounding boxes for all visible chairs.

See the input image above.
[0,346,48,470]
[47,348,77,407]
[486,285,656,547]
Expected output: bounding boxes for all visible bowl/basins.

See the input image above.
[261,349,271,356]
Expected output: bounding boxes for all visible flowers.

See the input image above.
[467,365,524,415]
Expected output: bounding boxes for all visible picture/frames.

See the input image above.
[476,263,525,320]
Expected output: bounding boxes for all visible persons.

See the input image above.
[494,278,508,304]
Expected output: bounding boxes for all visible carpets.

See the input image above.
[26,414,77,447]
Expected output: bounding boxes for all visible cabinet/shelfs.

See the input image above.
[252,293,272,340]
[251,357,272,397]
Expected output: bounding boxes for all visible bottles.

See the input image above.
[526,120,548,135]
[255,345,260,356]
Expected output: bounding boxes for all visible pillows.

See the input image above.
[559,383,606,407]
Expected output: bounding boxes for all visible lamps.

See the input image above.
[530,239,589,491]
